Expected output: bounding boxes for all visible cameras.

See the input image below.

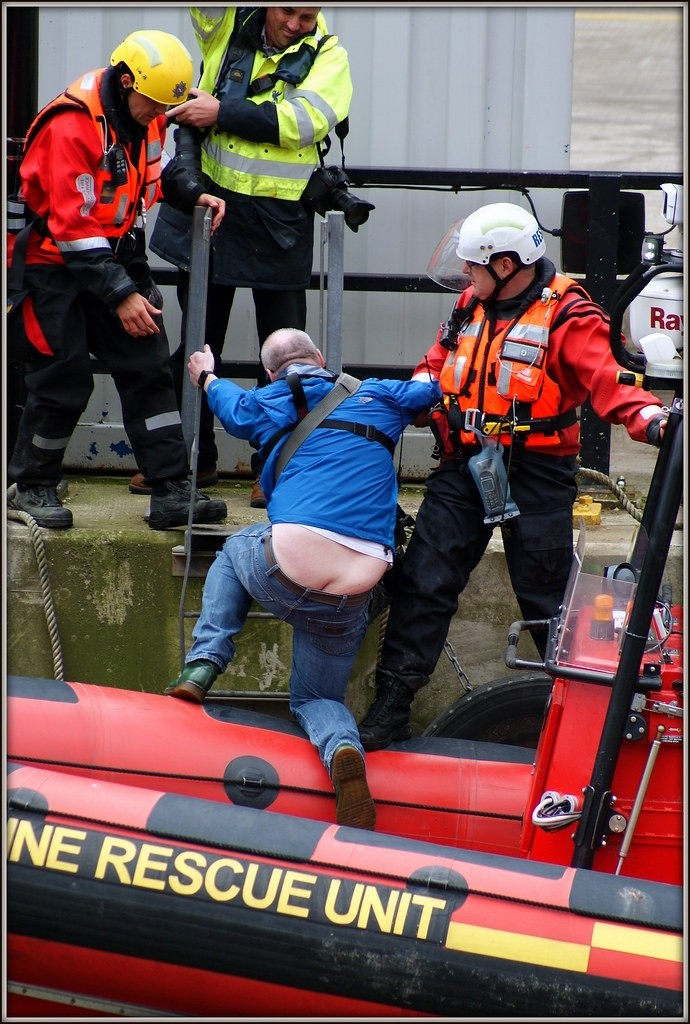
[300,166,376,233]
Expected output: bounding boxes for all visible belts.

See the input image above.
[264,536,385,608]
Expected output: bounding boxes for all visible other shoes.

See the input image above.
[148,480,227,528]
[250,474,269,508]
[10,484,73,528]
[128,461,219,493]
[355,693,413,750]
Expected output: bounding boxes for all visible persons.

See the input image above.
[357,201,668,756]
[5,27,228,530]
[161,325,443,833]
[127,5,354,506]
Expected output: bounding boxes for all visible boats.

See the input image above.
[4,393,687,1018]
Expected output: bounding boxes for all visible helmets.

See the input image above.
[111,30,193,105]
[455,202,546,265]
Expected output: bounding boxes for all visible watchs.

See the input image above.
[197,370,214,390]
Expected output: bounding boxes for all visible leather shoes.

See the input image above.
[164,660,218,702]
[331,743,376,831]
